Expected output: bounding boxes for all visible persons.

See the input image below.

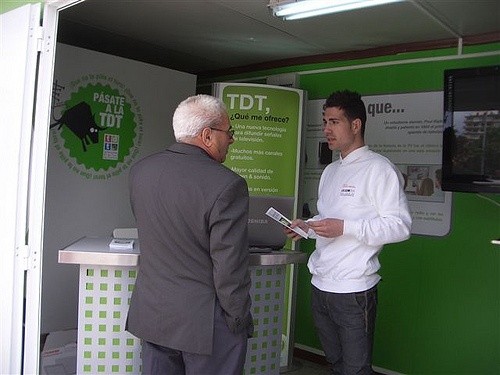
[125,95,254,375]
[283,90,412,375]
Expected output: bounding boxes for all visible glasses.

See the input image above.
[211,128,234,139]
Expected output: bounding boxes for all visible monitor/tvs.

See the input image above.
[441,66,500,194]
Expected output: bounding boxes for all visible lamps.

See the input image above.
[267,0,380,21]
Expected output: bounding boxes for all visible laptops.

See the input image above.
[247,197,294,250]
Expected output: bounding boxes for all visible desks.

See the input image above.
[57,235,309,375]
